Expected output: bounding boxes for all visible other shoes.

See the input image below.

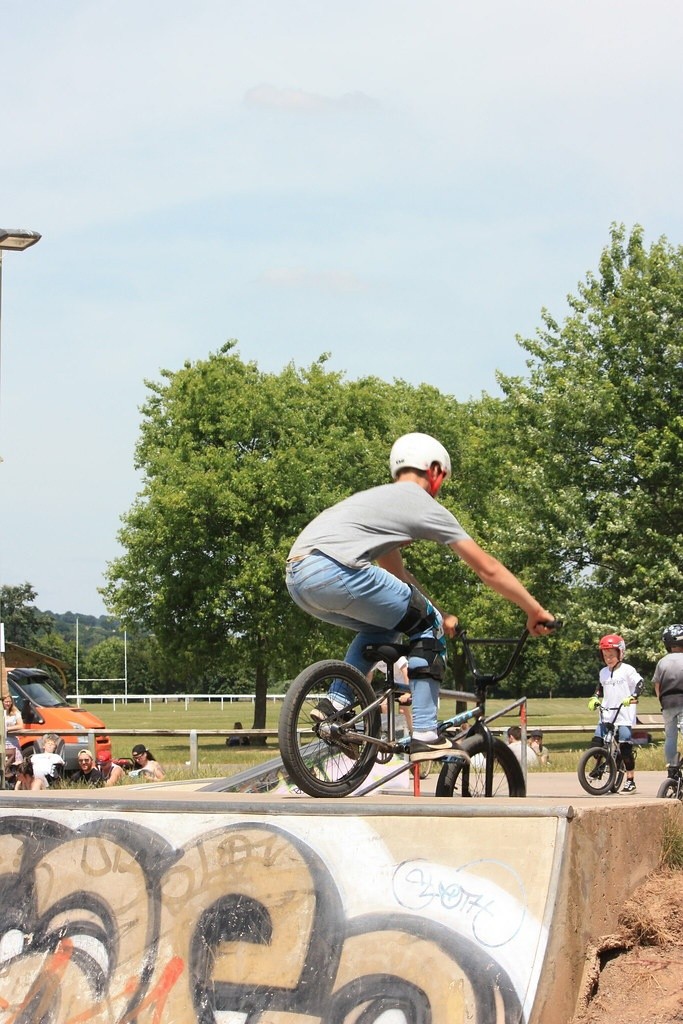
[669,791,683,800]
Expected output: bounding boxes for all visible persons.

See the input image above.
[529,729,553,768]
[94,751,125,787]
[588,634,644,795]
[31,738,65,787]
[13,757,47,790]
[0,696,23,790]
[225,722,250,746]
[365,633,413,740]
[126,744,167,782]
[443,723,486,769]
[506,726,540,766]
[651,624,683,789]
[286,431,555,762]
[67,749,104,788]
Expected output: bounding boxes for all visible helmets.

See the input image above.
[389,433,451,480]
[663,623,683,651]
[599,635,625,661]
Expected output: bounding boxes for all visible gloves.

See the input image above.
[587,697,600,712]
[622,696,635,707]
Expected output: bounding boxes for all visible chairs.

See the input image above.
[31,734,68,782]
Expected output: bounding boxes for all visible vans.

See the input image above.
[5,665,112,778]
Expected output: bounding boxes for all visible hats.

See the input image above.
[531,730,543,737]
[78,749,93,761]
[96,751,112,762]
[132,745,146,757]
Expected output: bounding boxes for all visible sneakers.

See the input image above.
[410,722,472,765]
[619,780,637,795]
[309,697,360,761]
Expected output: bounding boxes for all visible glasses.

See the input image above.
[133,753,143,761]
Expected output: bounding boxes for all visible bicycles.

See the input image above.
[656,751,683,800]
[277,619,564,798]
[372,689,434,779]
[577,699,641,796]
